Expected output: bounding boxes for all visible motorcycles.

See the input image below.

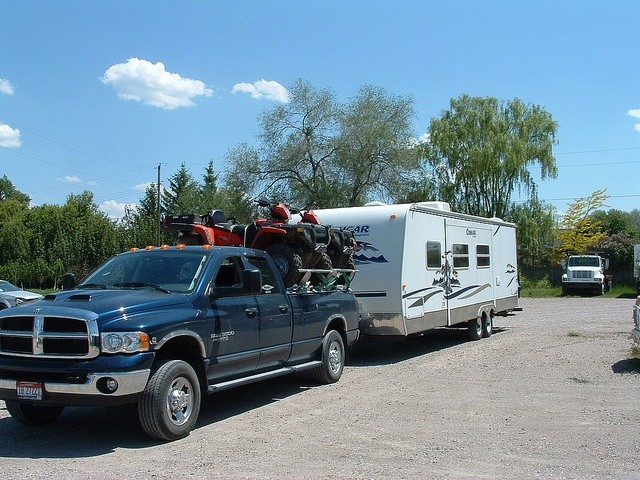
[199,200,357,286]
[159,198,332,289]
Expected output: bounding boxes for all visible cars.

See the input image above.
[0,280,42,310]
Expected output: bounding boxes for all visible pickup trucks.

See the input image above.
[0,245,360,441]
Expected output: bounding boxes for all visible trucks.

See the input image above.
[562,255,612,294]
